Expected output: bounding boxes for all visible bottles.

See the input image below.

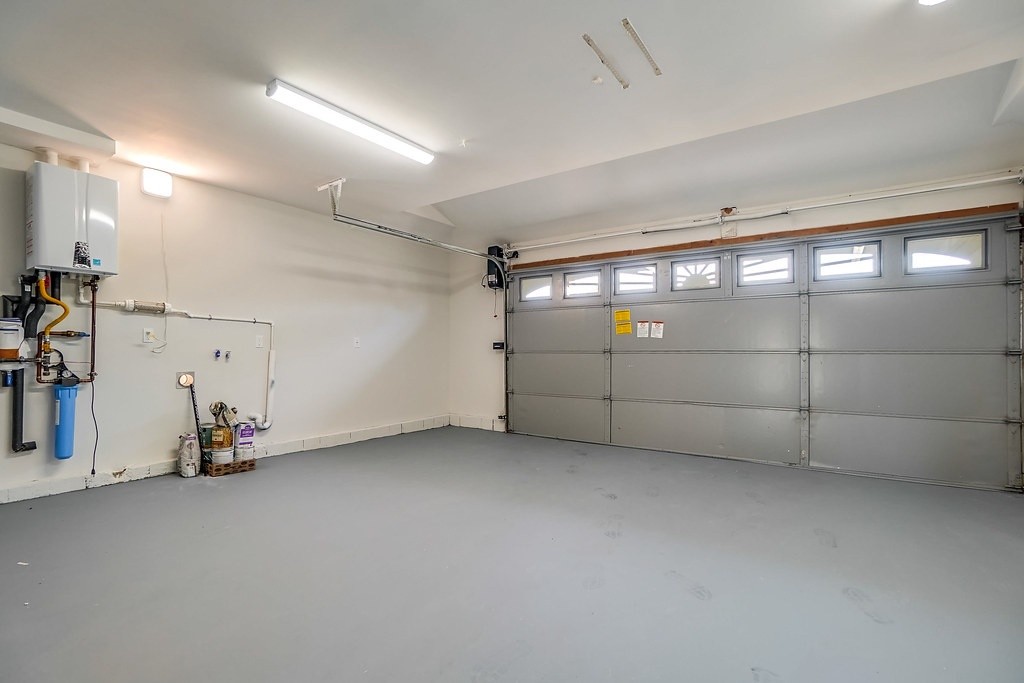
[176,432,201,478]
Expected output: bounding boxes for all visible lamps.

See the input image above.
[265,78,434,165]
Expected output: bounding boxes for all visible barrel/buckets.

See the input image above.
[200,420,255,464]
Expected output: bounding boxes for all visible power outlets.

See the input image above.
[143,328,154,343]
